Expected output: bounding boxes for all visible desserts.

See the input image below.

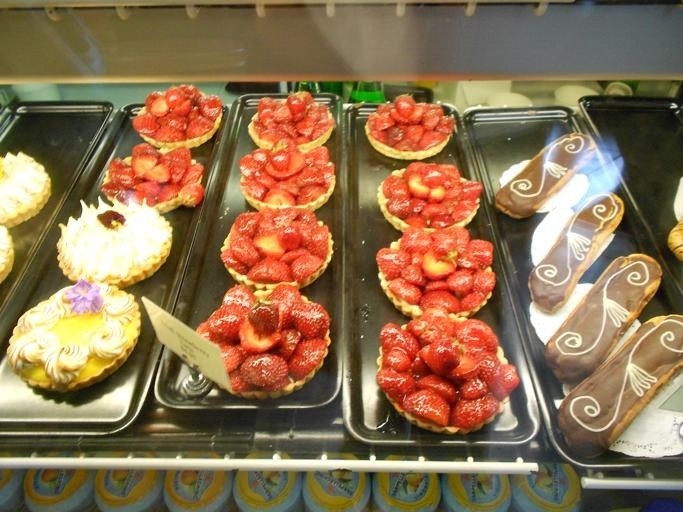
[1,151,173,396]
[493,133,683,456]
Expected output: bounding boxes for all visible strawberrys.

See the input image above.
[100,84,518,435]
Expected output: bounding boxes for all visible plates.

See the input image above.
[212,95,337,400]
